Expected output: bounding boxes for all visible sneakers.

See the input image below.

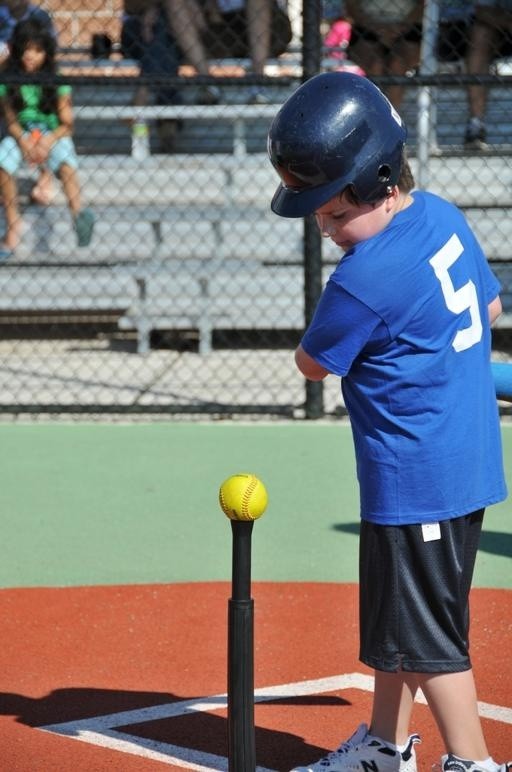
[289,722,512,772]
[464,122,489,150]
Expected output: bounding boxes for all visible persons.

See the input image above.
[133,2,295,107]
[321,1,428,117]
[434,0,511,153]
[0,0,60,63]
[0,13,99,252]
[260,64,510,772]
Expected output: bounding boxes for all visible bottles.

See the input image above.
[131,119,151,164]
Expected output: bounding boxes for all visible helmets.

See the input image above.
[266,71,408,218]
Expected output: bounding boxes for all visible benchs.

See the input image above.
[0,59,512,358]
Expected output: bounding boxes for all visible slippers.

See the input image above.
[74,208,95,247]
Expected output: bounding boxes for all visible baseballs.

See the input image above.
[219,474,267,521]
